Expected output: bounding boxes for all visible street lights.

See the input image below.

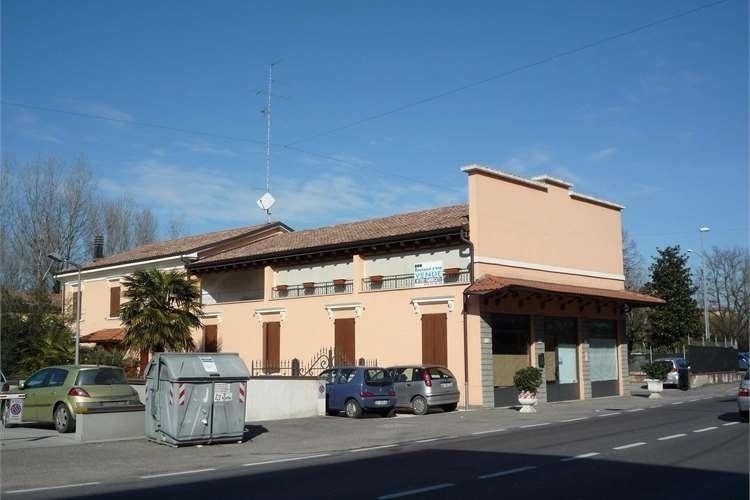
[46,251,83,366]
[685,223,713,345]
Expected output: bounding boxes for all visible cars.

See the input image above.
[0,370,9,418]
[737,351,750,370]
[736,366,750,412]
[3,364,144,433]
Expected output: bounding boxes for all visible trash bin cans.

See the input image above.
[143,352,250,448]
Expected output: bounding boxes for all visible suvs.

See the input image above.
[652,357,688,389]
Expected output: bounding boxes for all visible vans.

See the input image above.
[370,363,460,415]
[318,365,397,419]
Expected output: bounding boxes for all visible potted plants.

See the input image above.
[513,366,543,414]
[640,360,673,398]
